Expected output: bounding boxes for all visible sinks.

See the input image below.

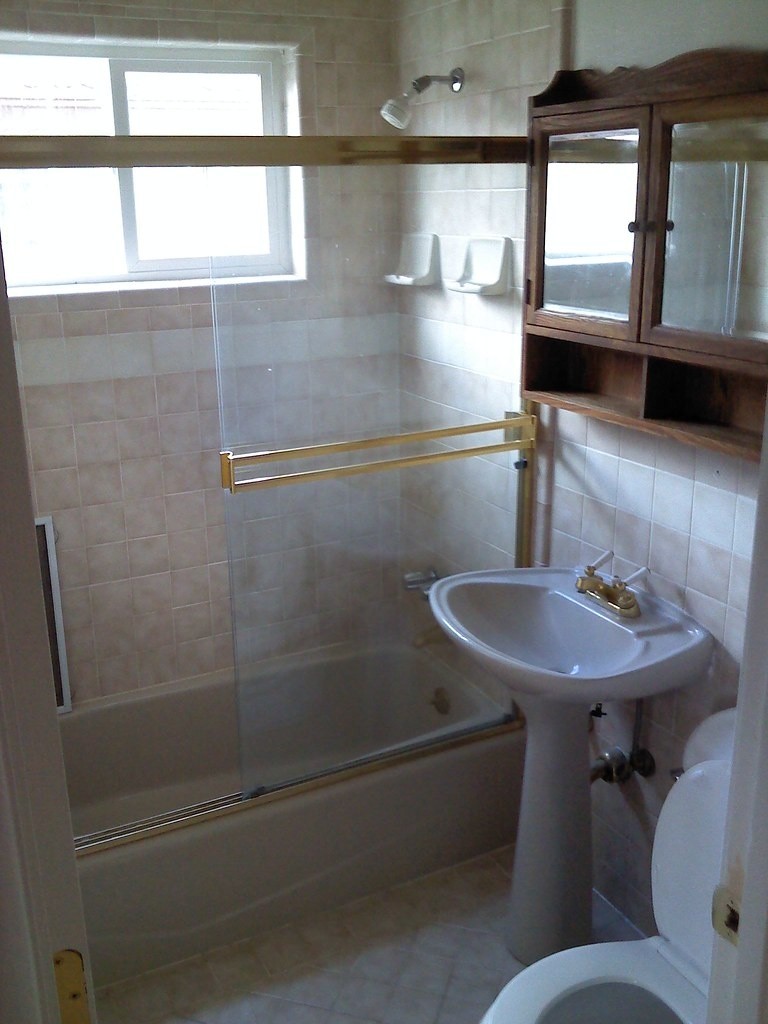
[429,565,717,714]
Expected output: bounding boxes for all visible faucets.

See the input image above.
[575,550,652,619]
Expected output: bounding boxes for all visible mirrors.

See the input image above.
[538,126,639,323]
[656,117,768,339]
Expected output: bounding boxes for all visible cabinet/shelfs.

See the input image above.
[519,47,768,460]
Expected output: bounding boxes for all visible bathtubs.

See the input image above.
[55,631,508,856]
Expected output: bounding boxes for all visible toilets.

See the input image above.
[478,757,733,1024]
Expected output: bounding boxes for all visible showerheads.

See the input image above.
[379,67,466,130]
